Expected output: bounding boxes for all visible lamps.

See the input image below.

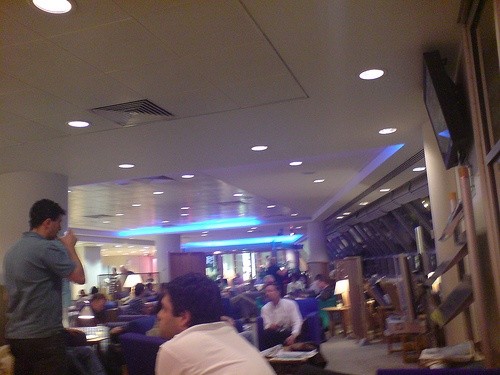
[123,271,144,299]
[332,278,350,305]
[78,299,96,319]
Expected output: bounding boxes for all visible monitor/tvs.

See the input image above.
[422,51,471,169]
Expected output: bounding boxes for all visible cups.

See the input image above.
[56,228,78,247]
[336,300,344,308]
[97,324,104,336]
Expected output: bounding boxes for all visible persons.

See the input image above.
[77,286,98,301]
[62,327,106,375]
[144,283,153,297]
[260,283,303,346]
[221,317,254,342]
[79,294,127,375]
[256,255,322,298]
[154,272,277,375]
[3,198,85,375]
[126,283,151,315]
[315,277,333,299]
[106,264,134,300]
[412,283,428,312]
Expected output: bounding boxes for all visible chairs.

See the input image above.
[255,297,325,350]
[120,319,245,375]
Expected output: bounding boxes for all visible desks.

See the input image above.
[322,305,354,337]
[71,325,116,351]
[384,332,418,363]
